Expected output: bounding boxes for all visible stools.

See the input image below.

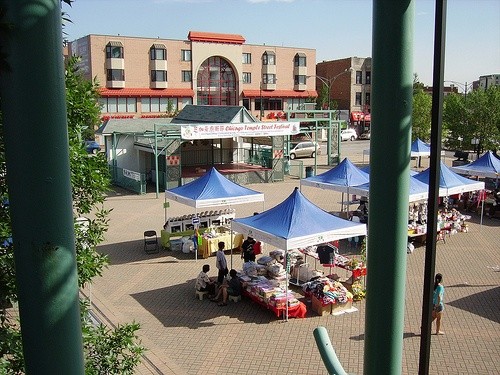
[195,290,208,300]
[229,295,241,303]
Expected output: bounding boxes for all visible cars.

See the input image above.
[283,141,321,159]
[82,141,101,154]
[359,129,370,139]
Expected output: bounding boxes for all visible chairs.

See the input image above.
[144,231,159,255]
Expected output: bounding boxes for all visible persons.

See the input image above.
[216,242,228,283]
[240,237,256,263]
[359,197,368,213]
[432,273,444,335]
[210,269,240,306]
[196,265,216,299]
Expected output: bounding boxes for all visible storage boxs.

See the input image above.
[305,292,352,316]
[170,240,182,250]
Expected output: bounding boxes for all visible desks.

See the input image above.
[408,215,471,244]
[160,228,216,250]
[197,230,244,259]
[240,281,308,318]
[297,248,367,287]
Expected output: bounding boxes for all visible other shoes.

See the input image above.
[218,303,227,306]
[210,298,218,302]
[436,330,445,335]
[208,294,215,298]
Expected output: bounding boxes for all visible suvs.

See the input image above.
[332,130,357,142]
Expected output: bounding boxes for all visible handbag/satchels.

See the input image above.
[197,234,202,246]
[222,269,228,275]
[253,240,262,255]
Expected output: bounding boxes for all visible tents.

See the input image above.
[450,150,500,190]
[164,167,265,260]
[412,161,486,224]
[230,187,368,321]
[300,157,369,217]
[410,138,429,167]
[348,177,429,220]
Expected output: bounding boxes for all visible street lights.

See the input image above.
[444,78,492,109]
[305,68,353,117]
